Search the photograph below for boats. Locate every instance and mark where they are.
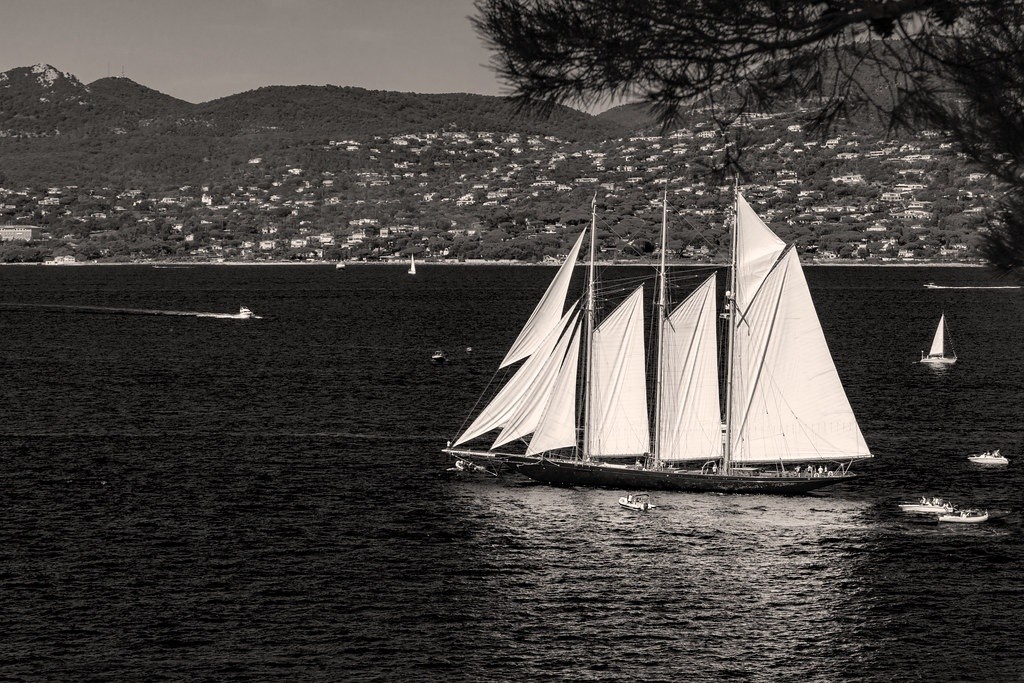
[335,261,347,271]
[238,304,257,319]
[431,350,446,362]
[967,448,1009,466]
[897,504,959,514]
[938,508,990,523]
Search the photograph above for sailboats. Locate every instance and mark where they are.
[407,252,417,275]
[438,171,877,496]
[919,313,958,364]
